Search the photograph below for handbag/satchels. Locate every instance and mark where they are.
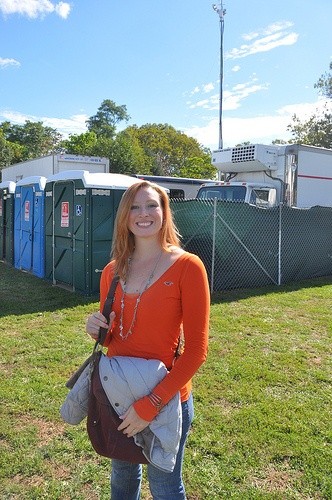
[88,353,147,462]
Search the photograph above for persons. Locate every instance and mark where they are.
[86,181,211,500]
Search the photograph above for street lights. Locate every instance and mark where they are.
[213,0,227,149]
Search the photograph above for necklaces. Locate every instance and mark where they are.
[119,249,163,341]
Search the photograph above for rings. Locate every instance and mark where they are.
[133,429,138,436]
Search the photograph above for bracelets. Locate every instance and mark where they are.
[147,391,165,412]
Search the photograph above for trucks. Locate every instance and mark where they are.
[170,143,332,286]
